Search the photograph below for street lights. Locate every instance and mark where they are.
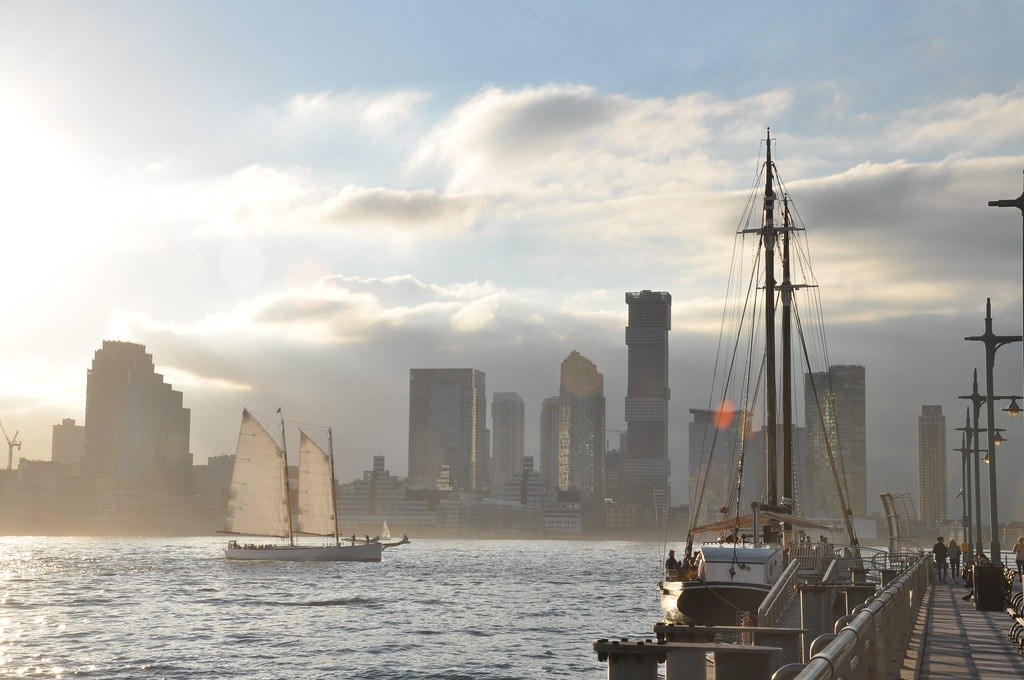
[954,406,1008,557]
[959,367,1024,563]
[951,432,989,555]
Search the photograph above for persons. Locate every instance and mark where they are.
[1012,537,1024,583]
[963,550,995,603]
[665,524,834,586]
[947,538,962,585]
[933,536,947,584]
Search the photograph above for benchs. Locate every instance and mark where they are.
[1006,592,1024,649]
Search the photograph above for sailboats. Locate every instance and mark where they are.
[215,404,414,564]
[648,127,875,627]
[379,519,391,540]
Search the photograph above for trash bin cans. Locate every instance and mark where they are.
[972,565,1004,611]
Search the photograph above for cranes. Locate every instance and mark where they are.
[1,418,24,472]
